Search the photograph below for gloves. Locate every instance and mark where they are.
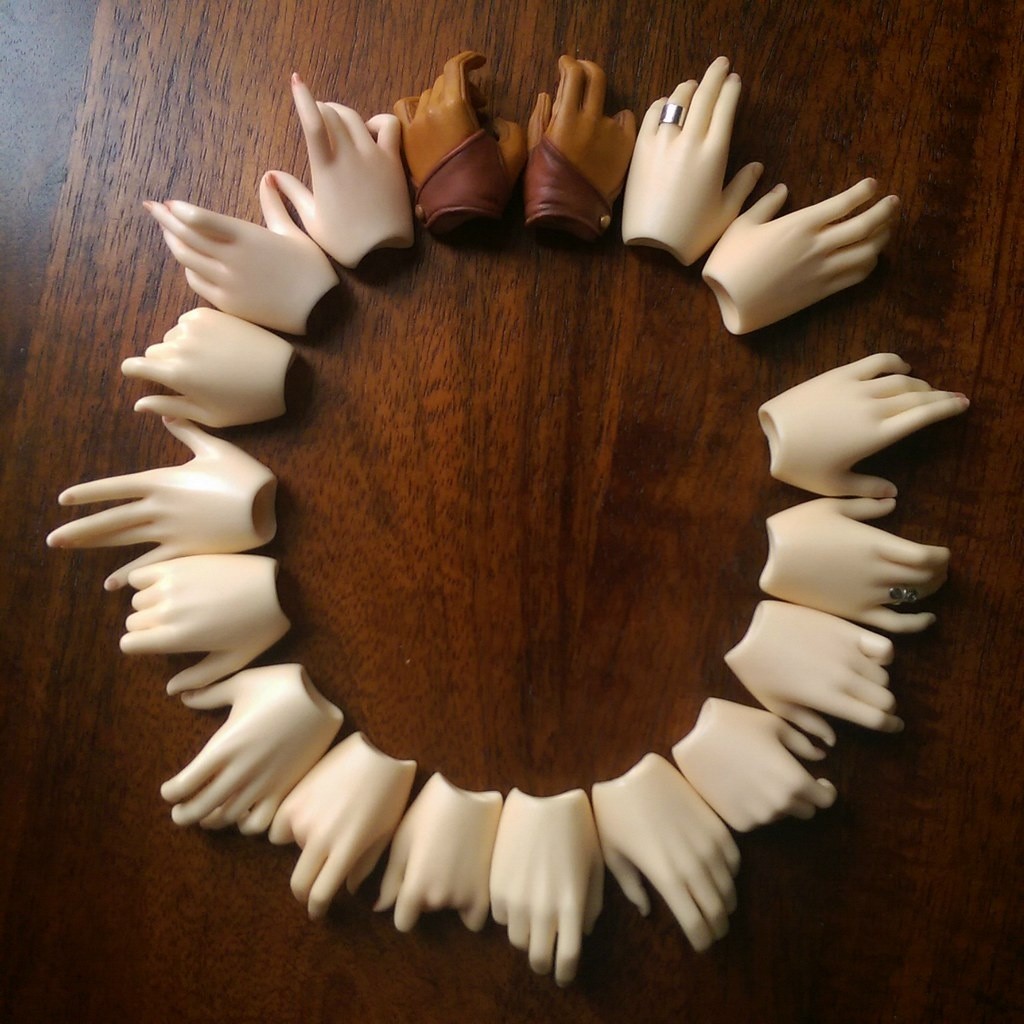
[524,55,636,243]
[393,51,525,245]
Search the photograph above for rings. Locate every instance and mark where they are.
[889,586,920,607]
[657,101,685,128]
